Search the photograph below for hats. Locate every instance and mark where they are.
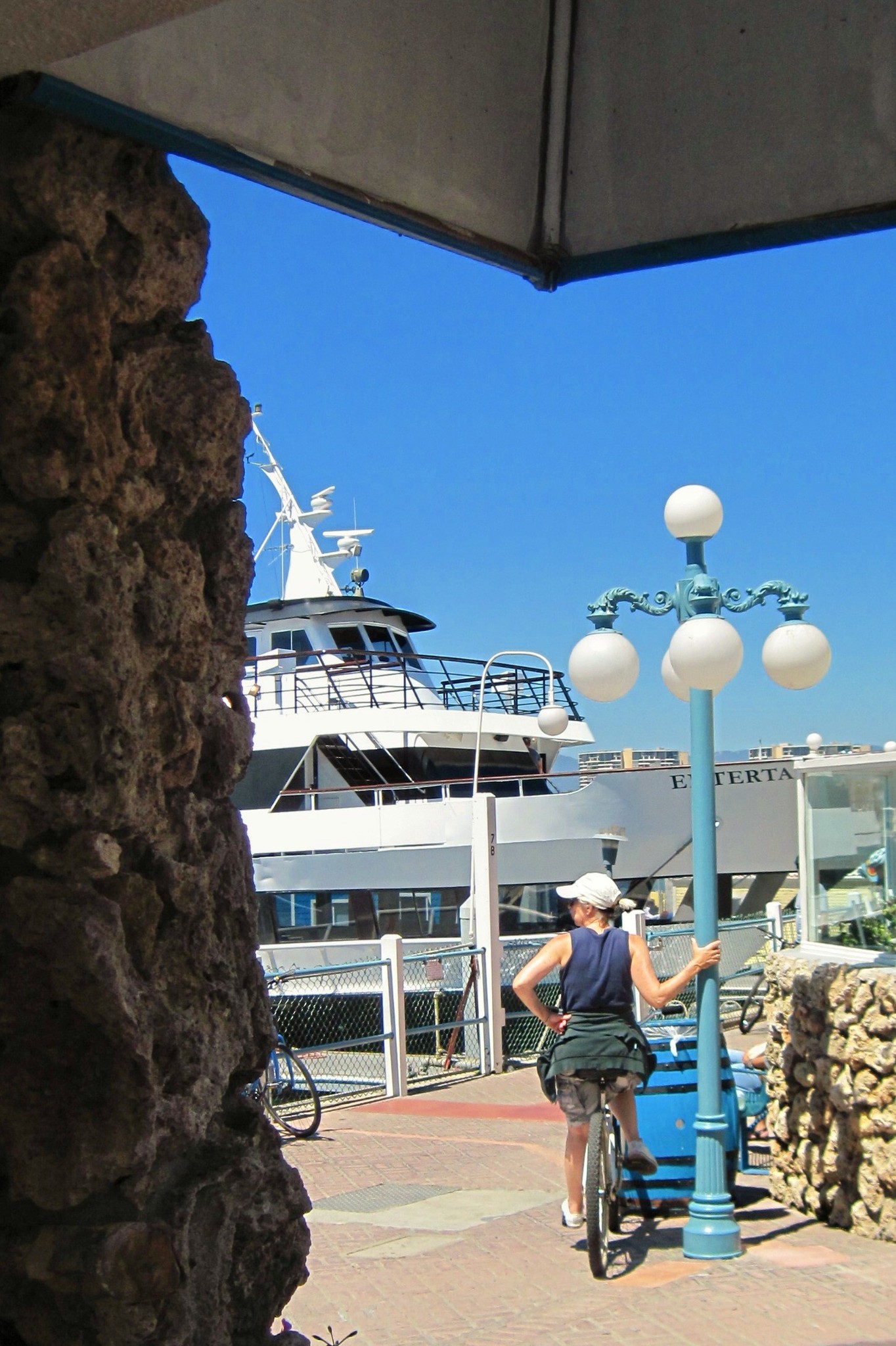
[556,872,622,910]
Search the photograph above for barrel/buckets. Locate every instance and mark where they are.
[617,1018,741,1208]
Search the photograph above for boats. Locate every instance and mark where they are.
[242,400,896,1057]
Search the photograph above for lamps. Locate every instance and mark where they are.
[350,568,370,597]
[495,734,508,742]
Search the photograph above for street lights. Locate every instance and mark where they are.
[565,483,834,1265]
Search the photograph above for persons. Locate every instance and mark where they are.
[643,899,668,920]
[742,1041,769,1140]
[512,872,722,1227]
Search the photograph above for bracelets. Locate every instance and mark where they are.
[748,1059,753,1069]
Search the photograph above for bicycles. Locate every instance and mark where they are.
[739,925,799,1035]
[544,1003,625,1281]
[239,969,322,1140]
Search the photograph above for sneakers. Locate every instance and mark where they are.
[562,1196,584,1227]
[626,1140,658,1175]
